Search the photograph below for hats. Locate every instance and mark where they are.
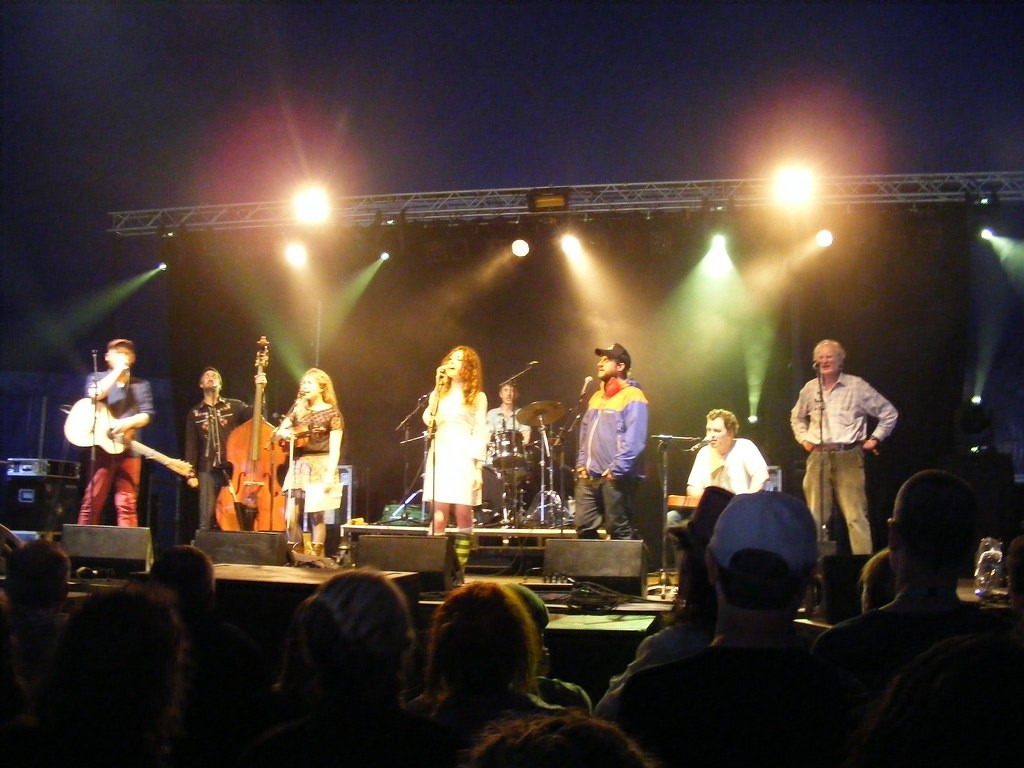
[595,342,631,369]
[710,489,819,571]
[669,484,732,556]
[107,338,134,348]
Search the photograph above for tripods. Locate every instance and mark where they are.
[387,371,575,526]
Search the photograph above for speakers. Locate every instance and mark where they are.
[0,475,77,532]
[60,523,154,576]
[355,534,463,591]
[805,555,878,626]
[543,540,648,598]
[194,528,291,567]
[464,546,544,575]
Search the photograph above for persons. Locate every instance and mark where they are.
[421,345,491,570]
[789,340,900,555]
[572,343,651,541]
[273,366,345,557]
[1,468,1024,768]
[79,338,155,528]
[184,367,269,533]
[667,408,770,568]
[488,379,531,446]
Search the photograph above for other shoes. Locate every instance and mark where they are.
[307,542,323,556]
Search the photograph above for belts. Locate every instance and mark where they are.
[813,443,857,451]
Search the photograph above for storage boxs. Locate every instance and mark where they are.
[7,458,81,479]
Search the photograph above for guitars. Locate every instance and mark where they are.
[63,398,193,477]
[667,495,701,509]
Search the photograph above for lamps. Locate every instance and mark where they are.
[964,194,1001,242]
[157,225,185,272]
[377,224,416,265]
[701,196,734,250]
[510,214,545,258]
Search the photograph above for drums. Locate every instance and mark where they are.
[449,464,506,528]
[486,442,504,475]
[494,429,526,472]
[382,504,431,527]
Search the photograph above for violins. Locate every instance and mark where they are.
[280,423,326,452]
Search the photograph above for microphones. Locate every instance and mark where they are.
[578,375,593,403]
[689,434,714,453]
[813,361,820,370]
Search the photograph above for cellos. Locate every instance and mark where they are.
[214,336,287,532]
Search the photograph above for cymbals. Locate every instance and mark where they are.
[516,401,565,427]
[525,437,562,450]
